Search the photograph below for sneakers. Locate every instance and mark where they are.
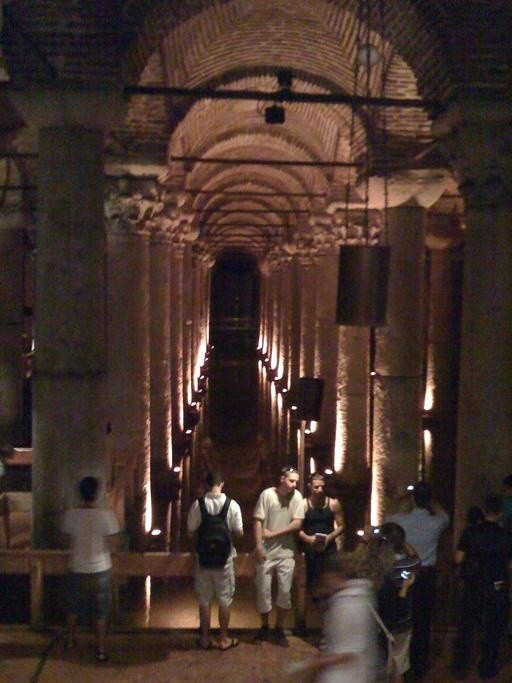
[254,625,288,647]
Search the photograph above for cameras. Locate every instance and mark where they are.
[400,570,409,578]
[372,527,381,534]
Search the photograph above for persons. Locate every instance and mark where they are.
[288,552,374,682]
[383,479,450,672]
[499,473,512,524]
[374,522,423,682]
[297,470,345,647]
[251,464,306,647]
[352,526,413,673]
[56,474,123,664]
[186,468,244,651]
[453,496,511,680]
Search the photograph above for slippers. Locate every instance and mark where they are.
[197,637,238,650]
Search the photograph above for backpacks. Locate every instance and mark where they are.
[196,495,231,568]
